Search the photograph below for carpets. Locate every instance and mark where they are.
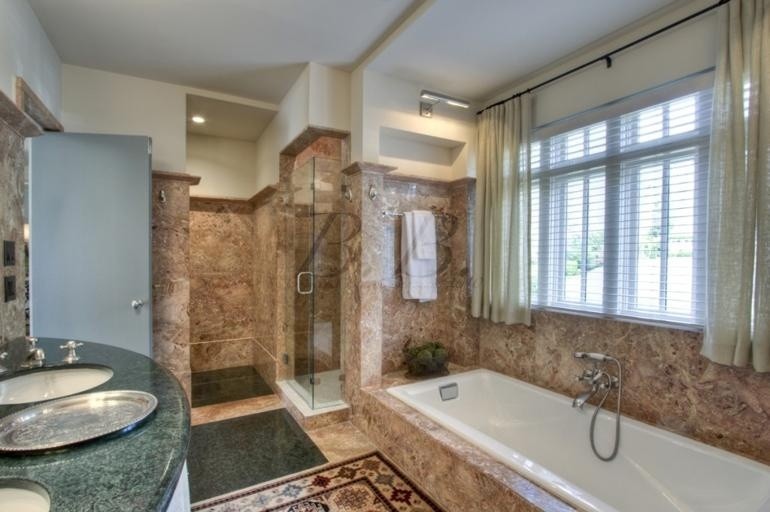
[182,404,451,512]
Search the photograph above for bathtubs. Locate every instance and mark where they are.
[310,319,332,366]
[385,365,770,511]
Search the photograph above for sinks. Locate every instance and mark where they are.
[0,363,115,406]
[0,476,52,512]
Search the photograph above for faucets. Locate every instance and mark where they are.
[21,335,47,368]
[571,381,600,409]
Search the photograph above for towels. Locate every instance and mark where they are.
[399,210,437,302]
